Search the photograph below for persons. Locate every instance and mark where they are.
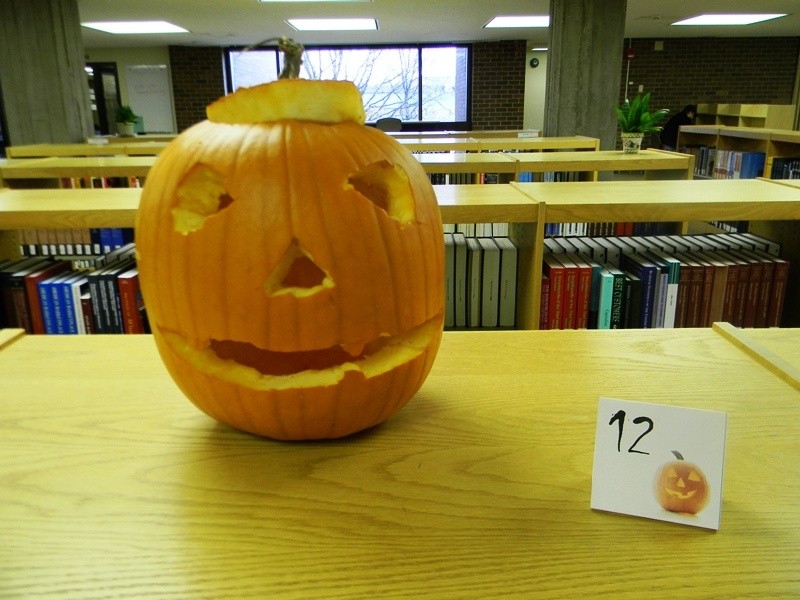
[659,104,697,153]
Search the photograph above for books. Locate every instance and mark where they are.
[0,128,800,336]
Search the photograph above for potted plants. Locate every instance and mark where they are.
[611,90,671,154]
[114,104,141,138]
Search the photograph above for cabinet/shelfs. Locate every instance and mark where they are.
[676,124,800,180]
[381,130,695,237]
[1,325,800,599]
[426,178,800,331]
[0,131,178,264]
[695,102,796,131]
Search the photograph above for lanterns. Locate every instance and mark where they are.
[655,450,709,516]
[132,37,451,442]
